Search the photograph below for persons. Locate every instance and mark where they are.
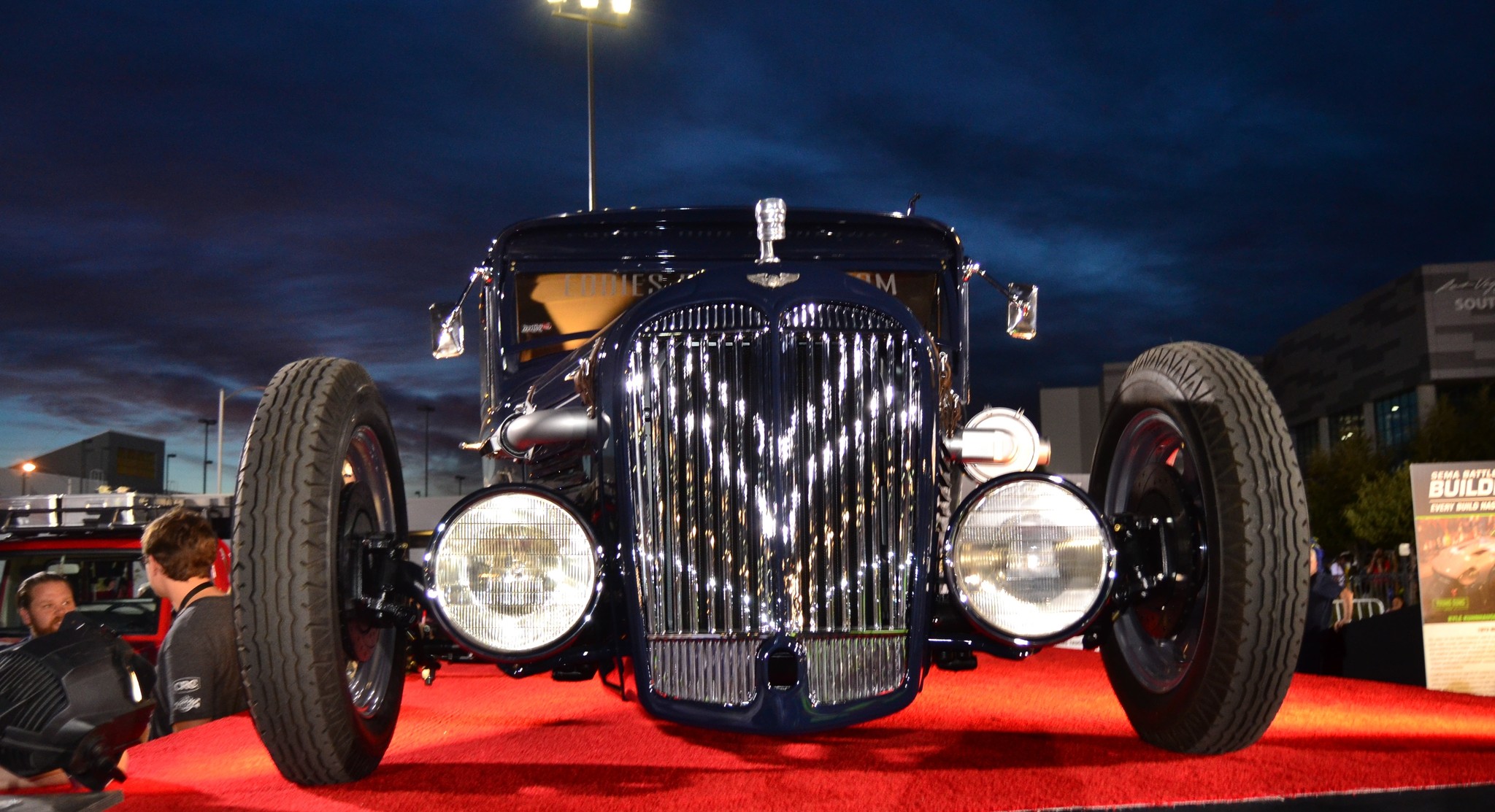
[1302,544,1419,646]
[1,571,77,651]
[141,509,251,742]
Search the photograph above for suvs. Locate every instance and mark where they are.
[0,493,235,679]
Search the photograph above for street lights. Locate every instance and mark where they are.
[165,453,177,492]
[198,417,218,493]
[455,474,466,494]
[21,463,36,496]
[416,404,436,497]
[548,0,632,212]
[79,437,94,494]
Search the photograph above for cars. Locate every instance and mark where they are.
[236,190,1310,788]
